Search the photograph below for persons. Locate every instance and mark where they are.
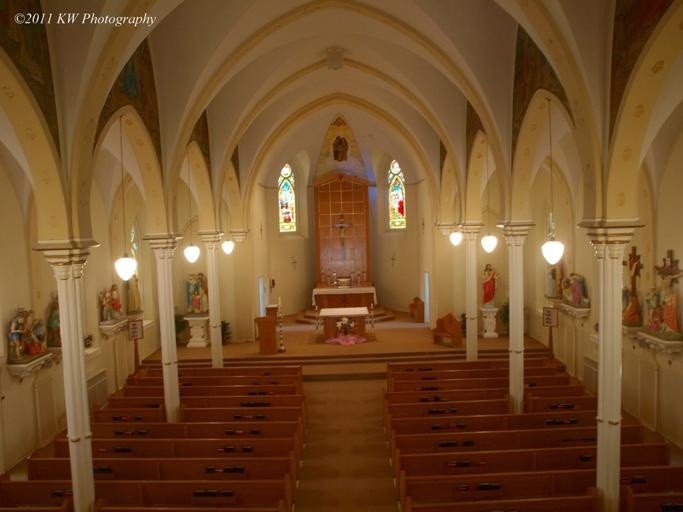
[622,254,682,336]
[481,263,501,308]
[184,272,209,314]
[561,273,586,306]
[6,290,61,363]
[333,225,353,246]
[101,283,124,321]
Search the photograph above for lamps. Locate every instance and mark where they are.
[538,100,564,267]
[183,150,234,266]
[447,142,498,256]
[112,117,137,283]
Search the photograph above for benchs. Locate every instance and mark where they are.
[0,362,310,512]
[381,354,682,511]
